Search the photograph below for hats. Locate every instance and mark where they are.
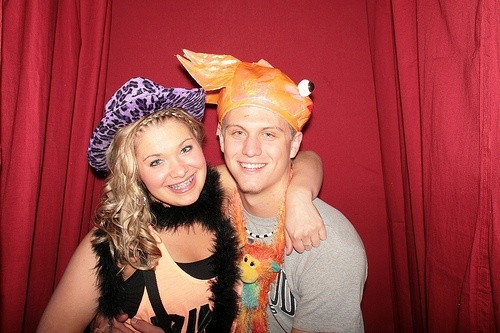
[87,76,207,172]
[176,49,315,132]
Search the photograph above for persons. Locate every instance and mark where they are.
[92,49,368,333]
[37,76,327,333]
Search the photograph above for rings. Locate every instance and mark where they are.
[304,242,312,246]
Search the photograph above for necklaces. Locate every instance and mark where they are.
[244,226,279,239]
